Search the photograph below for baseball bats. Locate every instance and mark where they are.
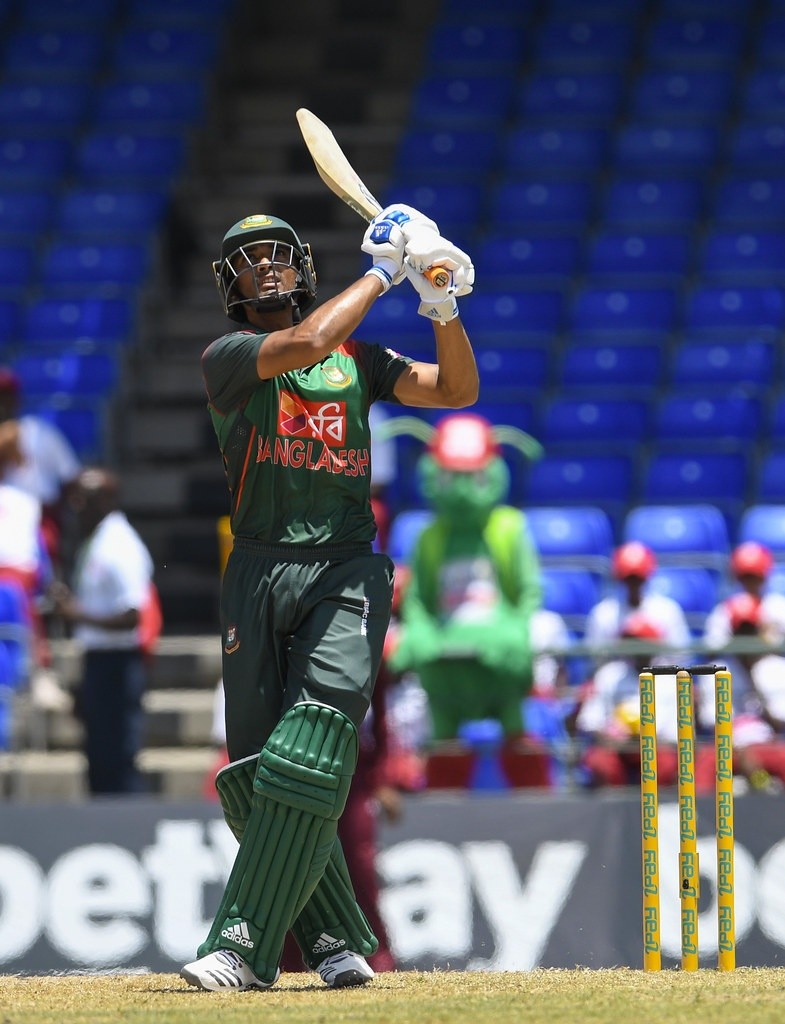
[295,107,452,290]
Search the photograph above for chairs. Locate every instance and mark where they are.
[0,0,784,789]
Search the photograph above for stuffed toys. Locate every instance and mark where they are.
[372,415,543,741]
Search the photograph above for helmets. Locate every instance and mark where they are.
[213,214,317,324]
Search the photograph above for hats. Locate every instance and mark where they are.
[611,540,656,578]
[731,541,774,578]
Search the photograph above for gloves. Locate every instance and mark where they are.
[404,217,475,326]
[360,203,440,297]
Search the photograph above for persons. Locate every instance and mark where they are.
[0,373,164,798]
[176,215,482,993]
[279,537,785,973]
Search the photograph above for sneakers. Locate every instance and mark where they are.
[180,949,282,993]
[316,949,374,986]
